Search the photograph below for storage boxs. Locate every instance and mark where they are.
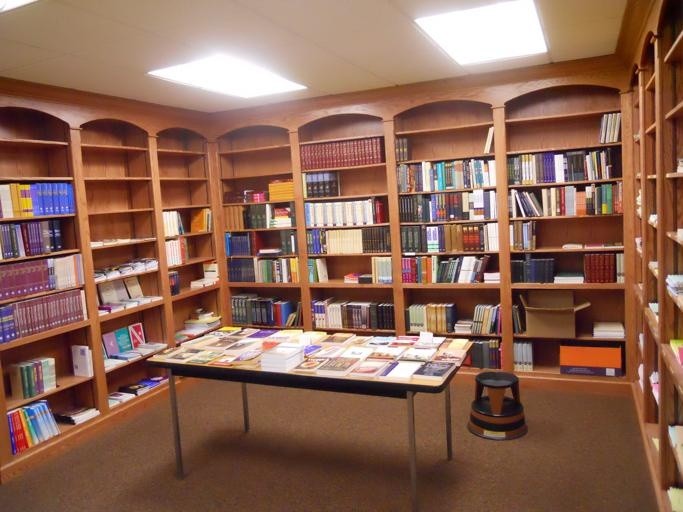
[559,342,622,377]
[519,290,591,339]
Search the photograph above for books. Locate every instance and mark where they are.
[394,127,497,224]
[162,207,220,297]
[506,112,624,282]
[299,137,395,330]
[634,159,683,512]
[592,320,625,338]
[222,179,303,327]
[90,238,169,408]
[400,222,501,336]
[147,308,501,382]
[514,342,533,372]
[0,183,89,344]
[6,345,101,455]
[512,303,523,335]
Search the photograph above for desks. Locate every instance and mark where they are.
[144,327,472,512]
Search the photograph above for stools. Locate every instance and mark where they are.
[467,372,528,441]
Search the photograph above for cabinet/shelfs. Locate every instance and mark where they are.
[210,56,631,397]
[614,0,683,512]
[0,77,227,484]
